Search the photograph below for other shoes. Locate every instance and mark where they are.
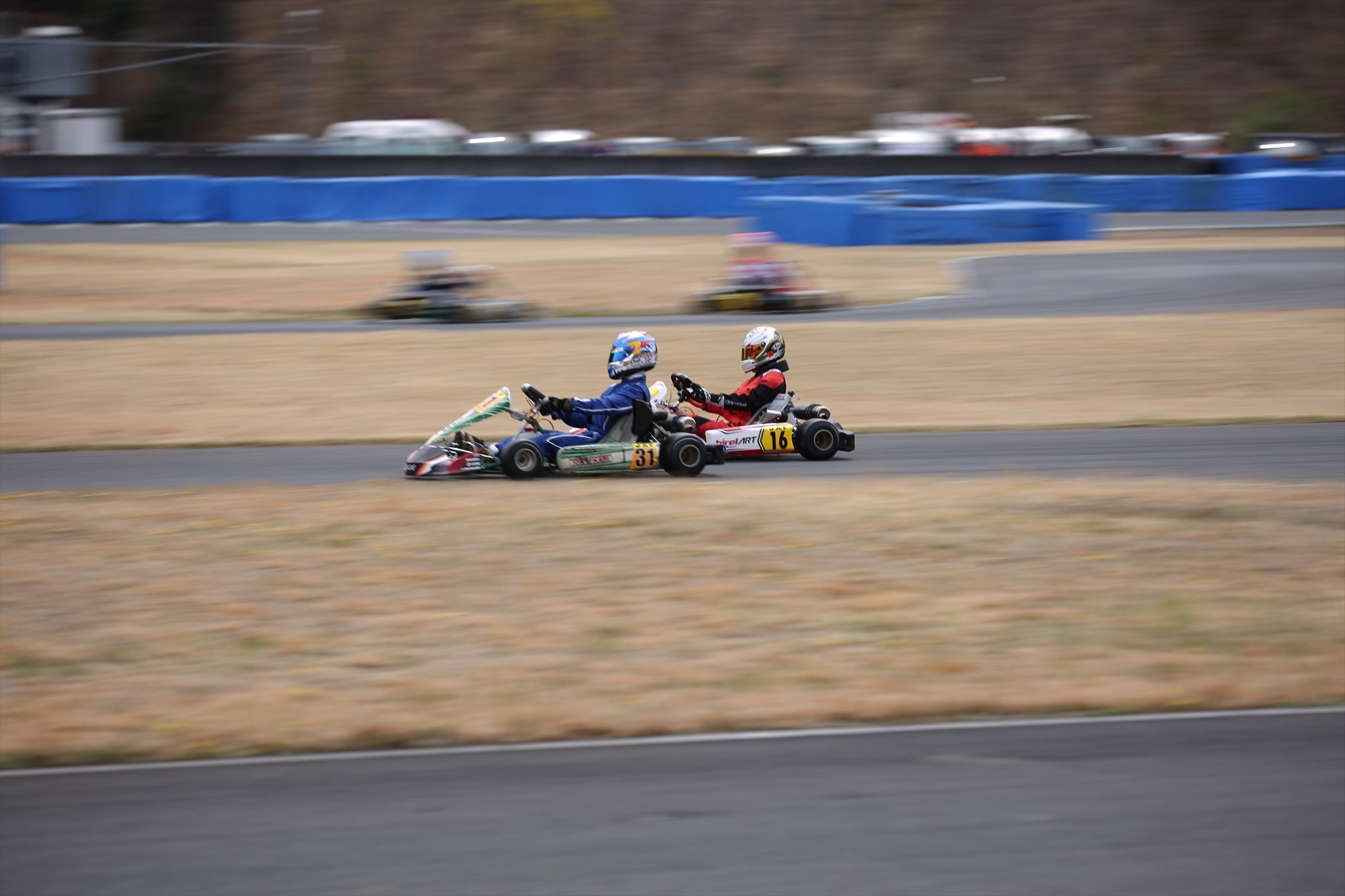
[455,430,468,457]
[465,432,487,455]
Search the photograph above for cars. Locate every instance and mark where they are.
[244,108,1345,160]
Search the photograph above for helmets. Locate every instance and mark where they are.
[740,326,784,373]
[605,330,658,379]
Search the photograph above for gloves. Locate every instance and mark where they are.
[539,396,571,416]
[689,387,711,403]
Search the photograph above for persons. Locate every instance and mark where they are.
[455,330,658,457]
[671,326,789,440]
